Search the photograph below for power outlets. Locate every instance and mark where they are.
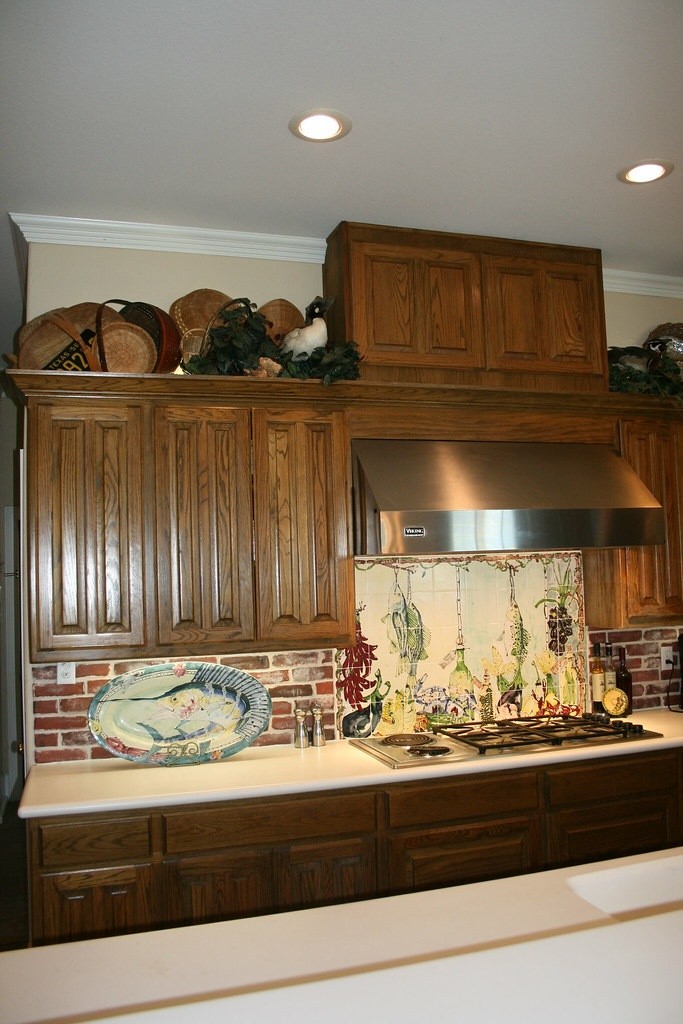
[661,646,673,670]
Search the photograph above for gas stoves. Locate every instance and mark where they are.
[347,712,665,769]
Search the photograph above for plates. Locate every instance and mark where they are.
[91,322,157,373]
[88,661,273,765]
[601,687,629,716]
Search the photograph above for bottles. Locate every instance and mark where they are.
[310,705,326,746]
[590,641,632,714]
[294,708,309,749]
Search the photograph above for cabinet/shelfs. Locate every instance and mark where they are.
[17,223,683,665]
[27,746,683,947]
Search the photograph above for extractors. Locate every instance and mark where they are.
[351,438,666,557]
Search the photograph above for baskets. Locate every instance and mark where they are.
[17,289,304,375]
